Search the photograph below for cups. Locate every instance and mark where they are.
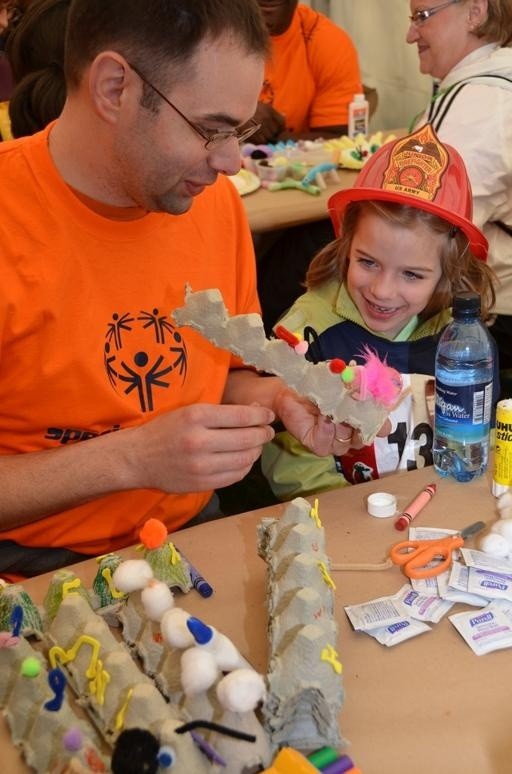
[240,152,316,184]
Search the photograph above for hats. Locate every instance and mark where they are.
[327,124,489,262]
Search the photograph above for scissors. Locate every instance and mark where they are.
[389,521,486,579]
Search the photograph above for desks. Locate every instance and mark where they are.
[221,127,416,239]
[0,460,512,772]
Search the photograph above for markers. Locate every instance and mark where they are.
[395,484,436,531]
[175,547,212,598]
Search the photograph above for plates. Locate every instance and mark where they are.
[227,168,262,197]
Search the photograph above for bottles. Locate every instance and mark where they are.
[432,286,494,481]
[348,91,369,141]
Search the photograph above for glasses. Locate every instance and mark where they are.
[409,0,456,25]
[127,61,262,150]
